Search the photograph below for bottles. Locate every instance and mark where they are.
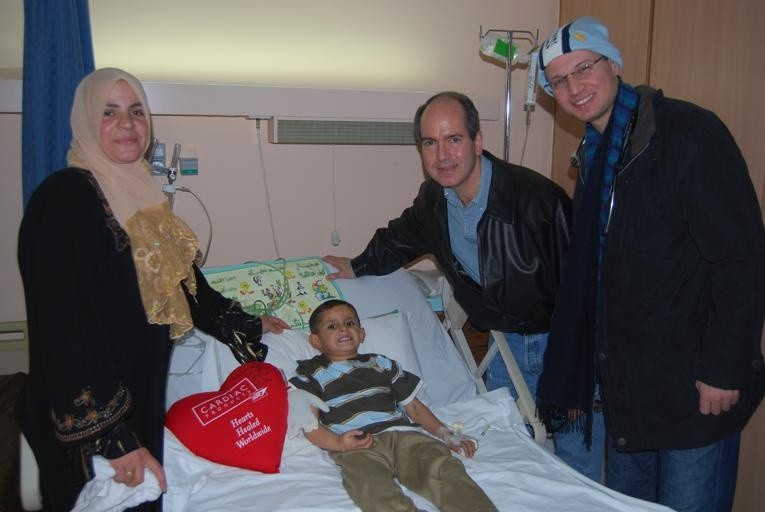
[479,33,530,68]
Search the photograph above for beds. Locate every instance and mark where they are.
[160,260,671,511]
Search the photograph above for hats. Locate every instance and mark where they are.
[537,16,622,96]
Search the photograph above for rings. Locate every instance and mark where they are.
[126,471,133,475]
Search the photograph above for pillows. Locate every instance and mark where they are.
[213,309,430,439]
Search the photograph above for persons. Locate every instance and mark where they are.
[322,93,606,487]
[533,18,764,511]
[14,68,289,511]
[291,300,497,512]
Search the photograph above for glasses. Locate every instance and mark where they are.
[544,57,604,96]
